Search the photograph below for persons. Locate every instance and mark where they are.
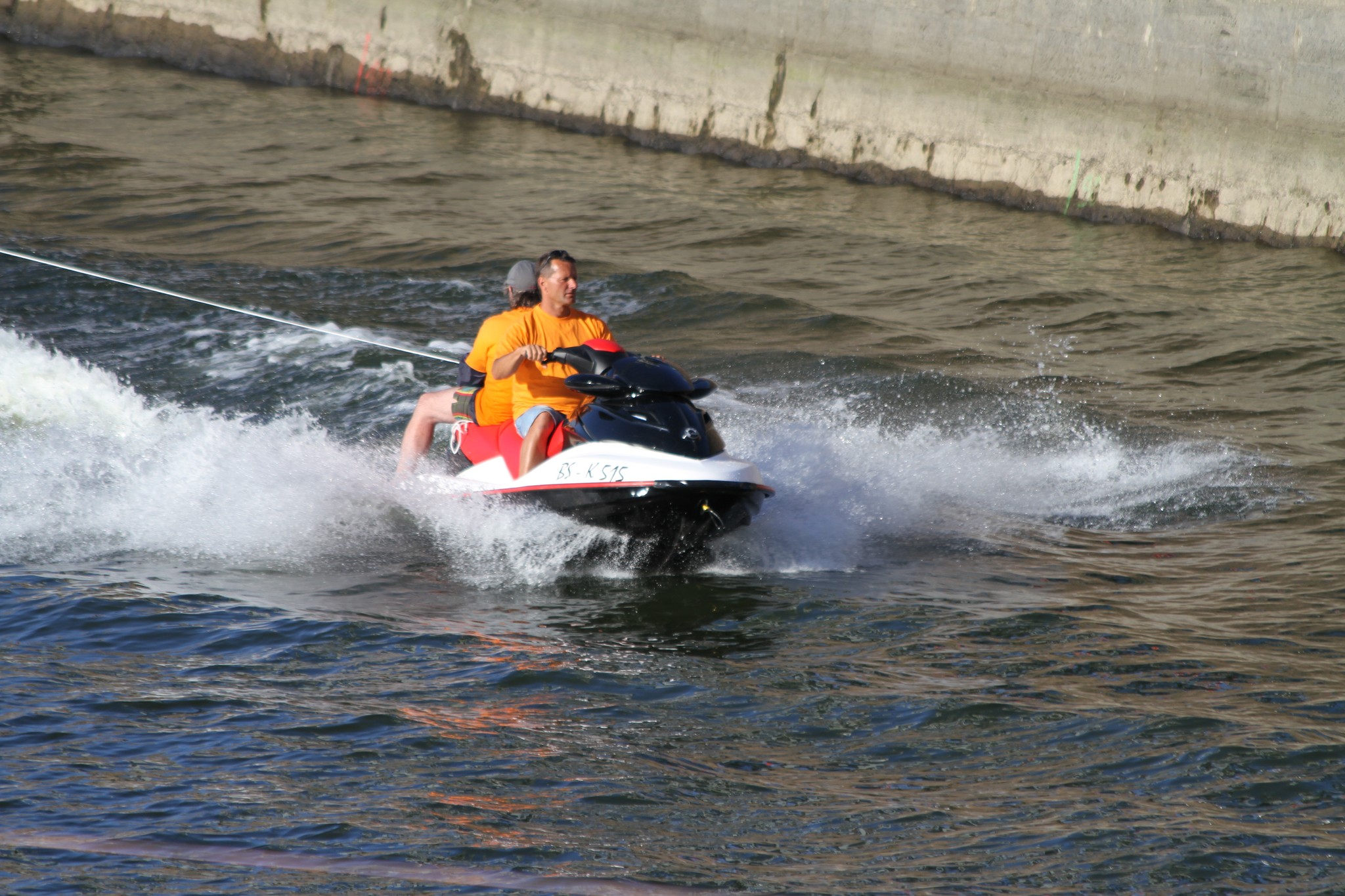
[395,259,543,485]
[487,249,615,481]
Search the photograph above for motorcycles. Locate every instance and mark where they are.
[400,338,780,580]
